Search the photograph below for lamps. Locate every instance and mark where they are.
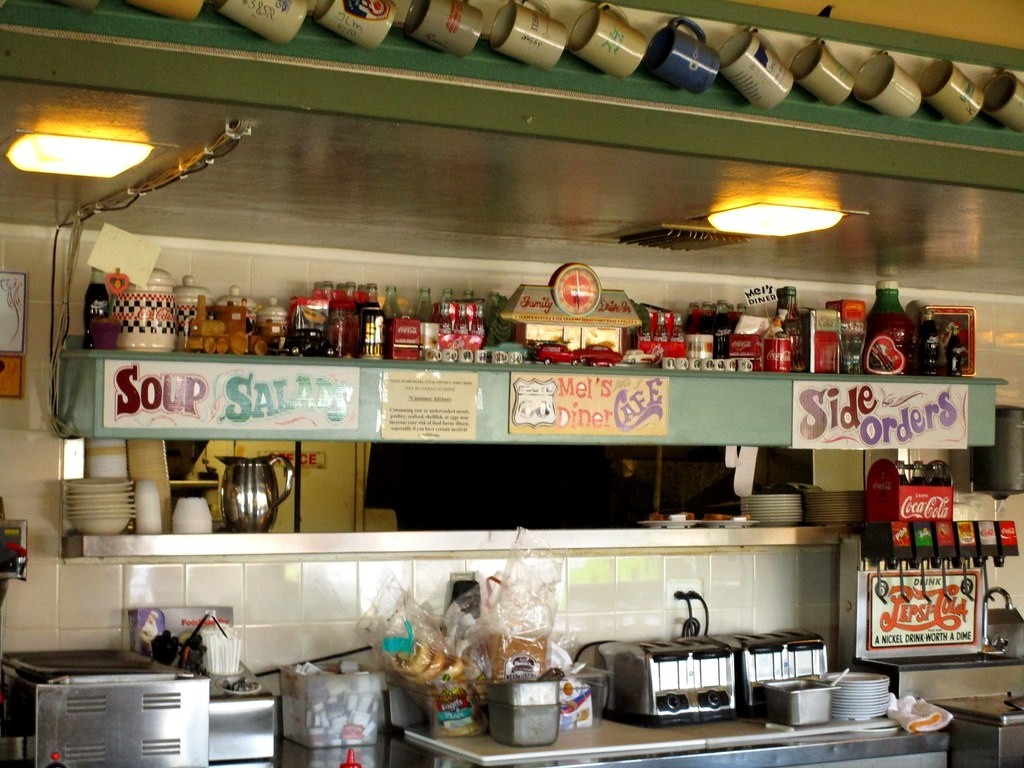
[0,130,178,178]
[685,201,869,238]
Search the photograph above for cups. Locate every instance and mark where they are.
[836,318,867,374]
[662,357,753,372]
[91,320,119,349]
[764,339,792,373]
[426,349,523,364]
[136,481,162,534]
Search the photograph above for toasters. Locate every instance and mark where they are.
[594,628,830,727]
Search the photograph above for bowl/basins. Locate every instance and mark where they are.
[63,438,172,532]
[173,497,213,534]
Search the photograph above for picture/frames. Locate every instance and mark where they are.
[923,306,977,376]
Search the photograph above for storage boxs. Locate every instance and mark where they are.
[127,605,234,658]
[560,680,593,730]
[278,666,384,747]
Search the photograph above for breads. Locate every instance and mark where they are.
[649,512,751,521]
[392,639,486,737]
[478,630,550,683]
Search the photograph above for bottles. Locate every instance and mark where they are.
[437,288,498,333]
[360,283,384,359]
[775,286,806,372]
[863,280,916,376]
[316,281,366,358]
[381,286,402,358]
[113,269,287,353]
[412,288,433,322]
[684,300,748,359]
[946,327,961,377]
[919,311,939,377]
[84,267,110,349]
[896,460,948,486]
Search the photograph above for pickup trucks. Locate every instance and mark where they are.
[623,350,656,365]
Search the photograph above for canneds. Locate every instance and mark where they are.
[358,306,386,360]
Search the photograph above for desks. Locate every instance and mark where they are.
[403,710,901,768]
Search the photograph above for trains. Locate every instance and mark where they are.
[186,295,286,356]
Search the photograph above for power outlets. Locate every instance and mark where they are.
[667,580,704,610]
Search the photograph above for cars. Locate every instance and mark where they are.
[483,343,532,360]
[271,328,336,358]
[532,344,582,366]
[575,344,622,368]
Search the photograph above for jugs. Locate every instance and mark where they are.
[215,455,293,533]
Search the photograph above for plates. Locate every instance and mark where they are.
[822,672,891,722]
[638,490,868,528]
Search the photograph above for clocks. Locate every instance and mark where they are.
[500,262,643,364]
